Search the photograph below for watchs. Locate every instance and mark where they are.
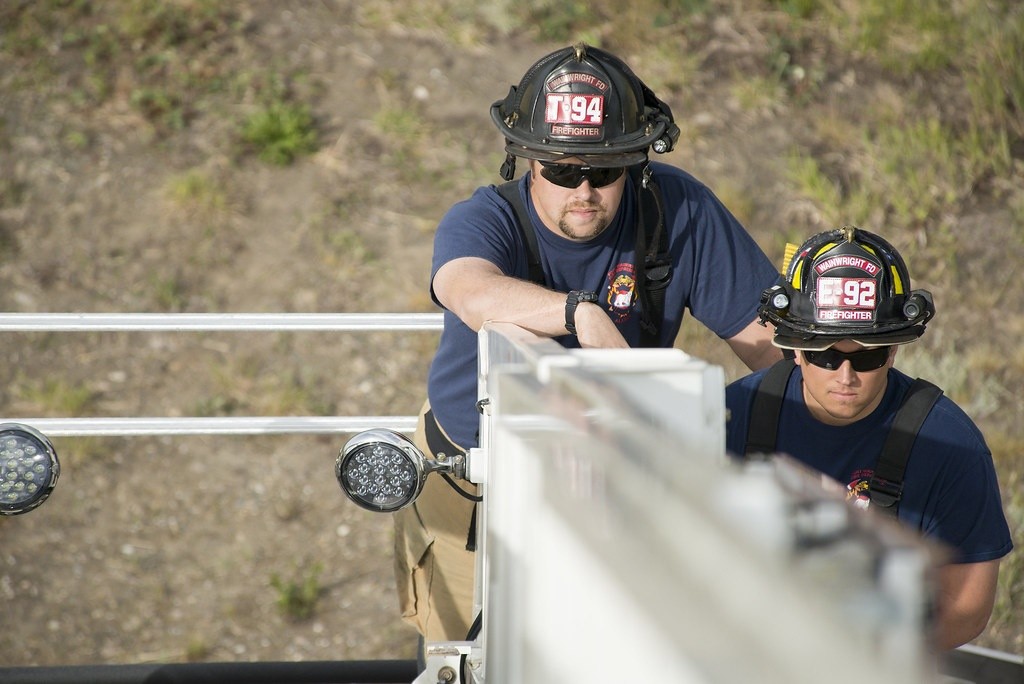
[564,290,598,335]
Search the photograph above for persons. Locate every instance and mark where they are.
[723,226,1014,656]
[396,45,789,684]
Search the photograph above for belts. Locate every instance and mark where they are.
[423,409,467,459]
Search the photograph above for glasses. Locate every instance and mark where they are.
[537,159,625,188]
[803,346,890,373]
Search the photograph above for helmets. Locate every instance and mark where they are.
[757,225,936,352]
[490,42,681,181]
